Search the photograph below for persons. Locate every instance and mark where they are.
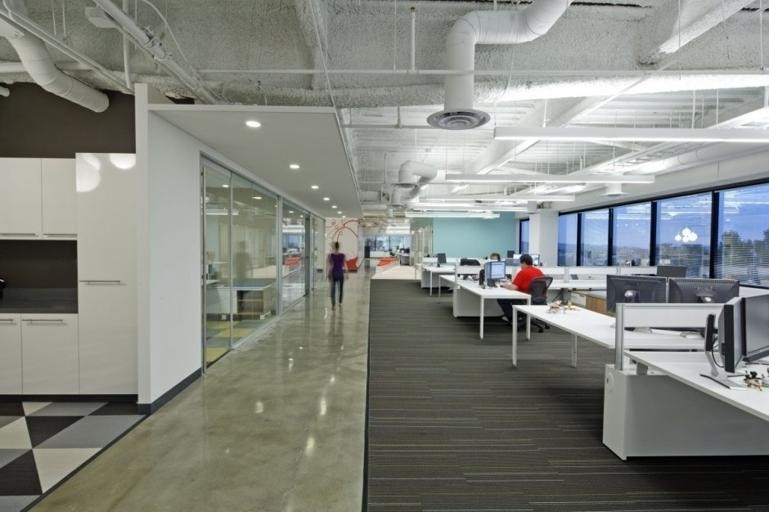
[324,242,349,310]
[233,240,255,311]
[490,253,500,262]
[497,253,545,328]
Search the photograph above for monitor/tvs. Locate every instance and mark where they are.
[607,275,667,335]
[461,250,540,281]
[656,265,687,278]
[437,253,446,267]
[700,297,747,390]
[668,277,740,338]
[742,294,769,357]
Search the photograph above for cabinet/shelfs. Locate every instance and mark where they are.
[0,157,77,241]
[75,152,139,394]
[0,312,79,395]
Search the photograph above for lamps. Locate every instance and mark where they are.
[405,90,768,220]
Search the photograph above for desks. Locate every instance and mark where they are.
[420,255,768,461]
